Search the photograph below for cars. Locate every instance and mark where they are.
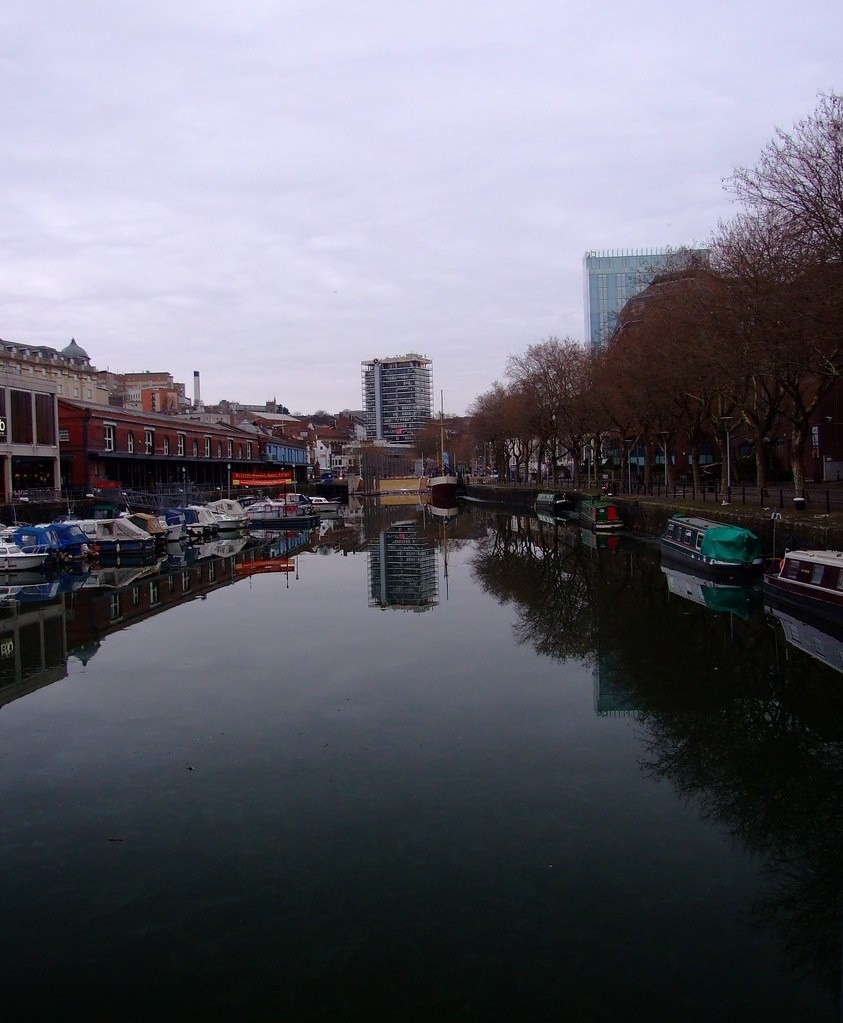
[169,483,197,494]
[321,473,336,484]
[490,470,498,478]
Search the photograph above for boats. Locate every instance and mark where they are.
[532,507,574,525]
[649,550,766,624]
[760,548,843,611]
[0,493,343,571]
[648,511,759,575]
[761,592,843,675]
[579,499,624,532]
[0,521,341,603]
[534,491,574,513]
[577,519,625,552]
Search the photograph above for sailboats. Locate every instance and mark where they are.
[426,408,459,496]
[426,496,461,600]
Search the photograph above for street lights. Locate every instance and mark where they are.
[547,450,552,483]
[586,446,592,490]
[183,467,185,492]
[720,416,737,503]
[228,463,231,498]
[291,462,296,493]
[626,440,635,494]
[657,431,672,497]
